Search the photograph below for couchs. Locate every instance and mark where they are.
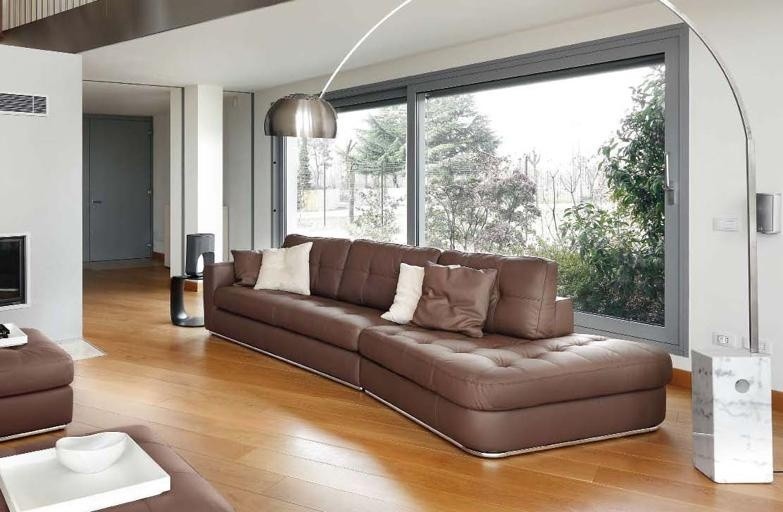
[1,328,75,441]
[2,423,228,512]
[203,228,672,458]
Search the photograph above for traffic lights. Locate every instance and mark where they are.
[230,242,314,295]
[380,258,498,339]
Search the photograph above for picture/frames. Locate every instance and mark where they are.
[691,349,773,486]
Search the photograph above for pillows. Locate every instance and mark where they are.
[230,242,314,295]
[380,258,498,339]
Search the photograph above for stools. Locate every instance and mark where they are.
[171,273,204,327]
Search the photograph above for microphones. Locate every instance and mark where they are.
[171,273,204,327]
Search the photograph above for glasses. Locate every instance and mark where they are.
[263,0,759,354]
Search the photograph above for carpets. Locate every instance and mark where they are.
[84,258,162,272]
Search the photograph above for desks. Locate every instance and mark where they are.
[691,349,773,486]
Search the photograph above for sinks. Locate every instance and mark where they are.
[203,228,672,458]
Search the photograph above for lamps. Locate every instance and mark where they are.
[263,0,759,354]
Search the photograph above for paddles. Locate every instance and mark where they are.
[84,258,162,272]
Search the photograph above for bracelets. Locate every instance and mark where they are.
[55,431,129,474]
